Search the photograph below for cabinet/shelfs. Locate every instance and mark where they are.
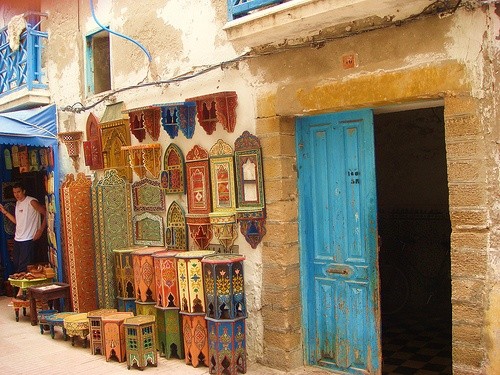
[7,277,51,322]
[28,282,71,334]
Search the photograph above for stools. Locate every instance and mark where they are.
[63,312,90,347]
[37,308,58,339]
[46,311,76,341]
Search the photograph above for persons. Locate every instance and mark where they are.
[0,183,48,307]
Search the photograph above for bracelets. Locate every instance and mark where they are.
[4,211,8,215]
